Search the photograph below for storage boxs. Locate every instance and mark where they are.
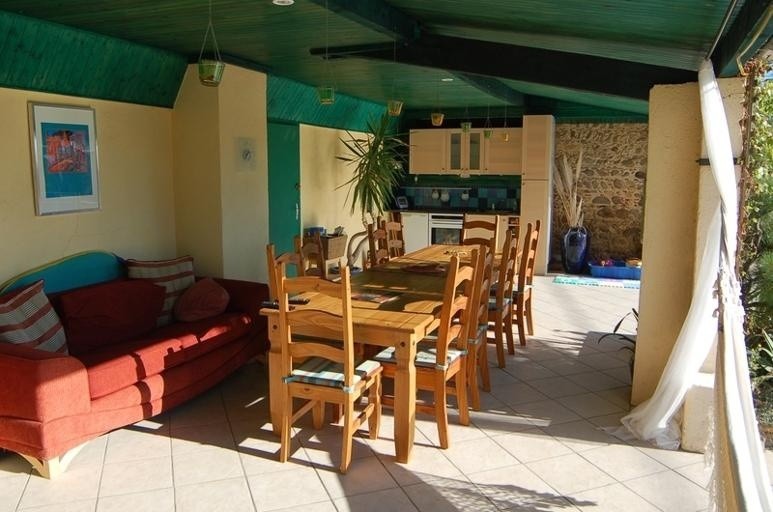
[587,260,634,279]
[302,233,348,260]
[625,262,641,280]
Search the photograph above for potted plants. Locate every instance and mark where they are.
[551,146,591,274]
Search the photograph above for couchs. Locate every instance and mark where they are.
[0,276,271,480]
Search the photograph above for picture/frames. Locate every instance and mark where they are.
[27,100,103,216]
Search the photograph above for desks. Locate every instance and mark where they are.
[258,242,523,464]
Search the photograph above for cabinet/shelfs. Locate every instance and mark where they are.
[309,257,342,282]
[521,115,555,180]
[409,128,523,175]
[384,212,428,255]
[465,214,521,274]
[517,181,553,275]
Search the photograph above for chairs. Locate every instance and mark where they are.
[489,220,541,346]
[370,249,479,449]
[420,238,496,411]
[487,226,522,368]
[460,212,499,250]
[276,261,384,474]
[266,236,307,302]
[377,214,405,264]
[368,220,391,268]
[295,231,326,293]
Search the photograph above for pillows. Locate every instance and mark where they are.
[127,254,196,328]
[47,279,167,353]
[0,278,70,355]
[173,278,230,323]
